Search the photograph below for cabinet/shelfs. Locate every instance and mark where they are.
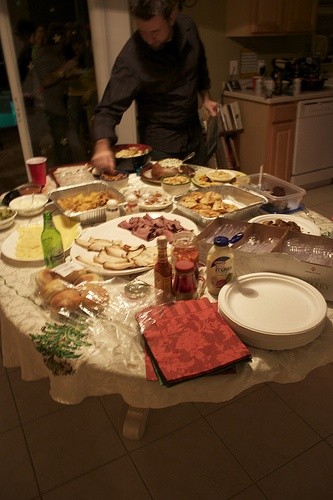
[222,92,333,187]
[225,0,320,37]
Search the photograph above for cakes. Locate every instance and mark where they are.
[151,158,184,178]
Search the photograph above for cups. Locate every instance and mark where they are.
[26,157,49,186]
[253,75,301,96]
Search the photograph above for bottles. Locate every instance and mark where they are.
[40,212,65,269]
[153,230,243,303]
[126,195,139,217]
[106,200,120,222]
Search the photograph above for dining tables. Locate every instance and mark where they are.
[0,158,333,438]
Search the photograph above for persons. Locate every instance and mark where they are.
[24,34,85,163]
[92,0,221,175]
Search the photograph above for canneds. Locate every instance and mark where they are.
[172,232,201,284]
[292,78,301,96]
[252,75,264,95]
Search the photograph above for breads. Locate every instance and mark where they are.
[178,192,240,221]
[34,270,111,315]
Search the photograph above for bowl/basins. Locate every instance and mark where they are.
[170,184,269,228]
[112,143,153,171]
[150,158,183,178]
[46,180,131,226]
[236,172,306,212]
[101,169,129,188]
[0,212,18,231]
[9,194,50,217]
[207,171,235,183]
[161,174,191,196]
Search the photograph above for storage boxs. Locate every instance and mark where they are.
[191,214,333,301]
[240,171,305,210]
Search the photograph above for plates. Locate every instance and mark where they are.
[69,211,196,276]
[142,165,197,183]
[1,220,81,262]
[247,214,321,239]
[192,169,250,188]
[120,184,173,209]
[217,272,327,351]
